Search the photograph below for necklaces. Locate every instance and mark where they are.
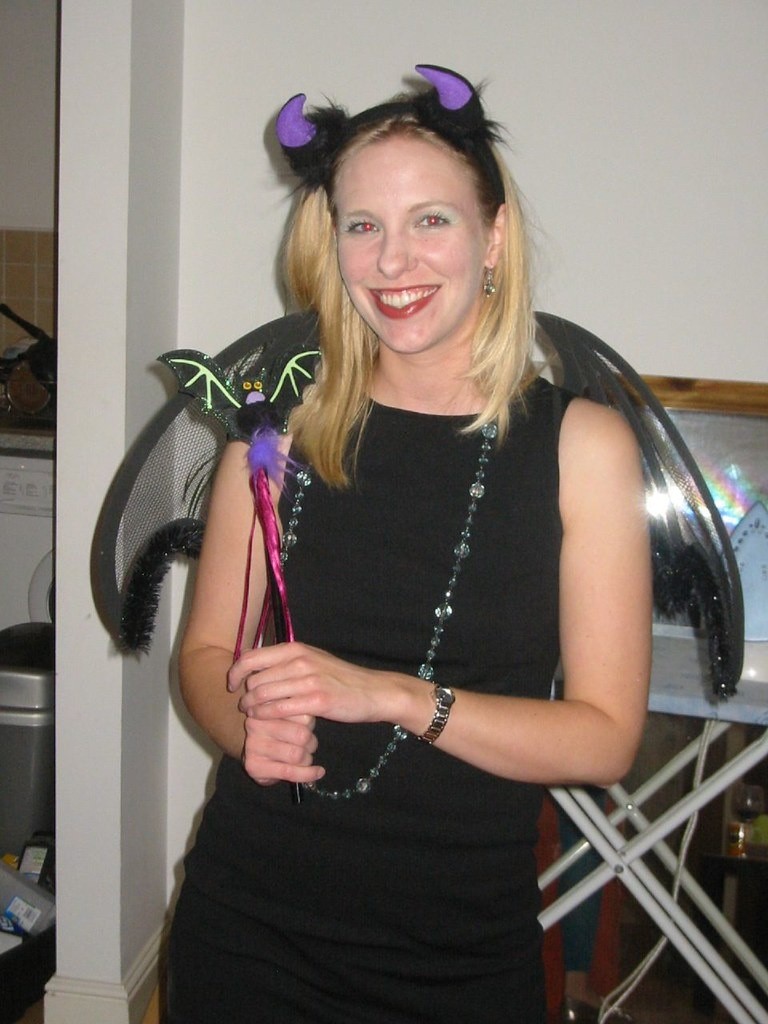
[277,420,499,800]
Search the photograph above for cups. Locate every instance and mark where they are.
[727,822,748,855]
[733,784,765,822]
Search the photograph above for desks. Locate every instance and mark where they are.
[538,635,768,1024]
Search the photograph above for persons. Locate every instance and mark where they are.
[158,64,654,1024]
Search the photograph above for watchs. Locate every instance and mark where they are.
[412,681,456,745]
